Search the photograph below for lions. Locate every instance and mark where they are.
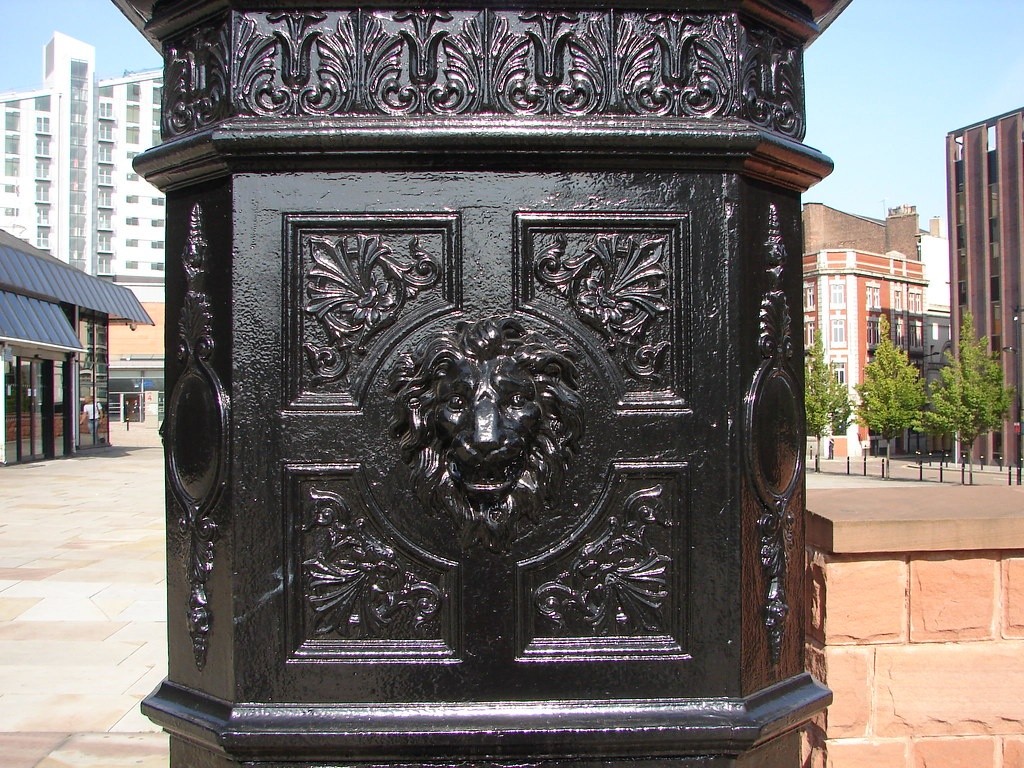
[380,314,587,557]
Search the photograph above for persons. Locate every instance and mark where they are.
[828,438,834,459]
[79,394,103,443]
